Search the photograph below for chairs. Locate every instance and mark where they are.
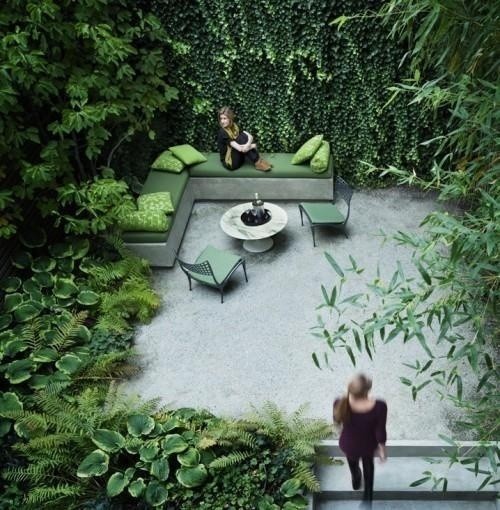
[298,176,355,249]
[171,243,248,303]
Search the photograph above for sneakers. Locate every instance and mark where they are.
[255,159,272,171]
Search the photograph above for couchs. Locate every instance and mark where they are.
[117,151,334,269]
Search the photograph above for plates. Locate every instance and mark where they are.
[240,207,273,227]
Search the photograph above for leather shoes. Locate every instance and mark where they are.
[361,498,372,509]
[352,468,362,490]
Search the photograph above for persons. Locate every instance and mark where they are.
[217,106,272,171]
[332,372,387,510]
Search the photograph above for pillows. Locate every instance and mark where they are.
[117,209,170,233]
[150,149,186,174]
[169,143,208,167]
[135,192,174,214]
[309,139,330,173]
[291,134,324,164]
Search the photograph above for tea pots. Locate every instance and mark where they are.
[251,192,267,220]
